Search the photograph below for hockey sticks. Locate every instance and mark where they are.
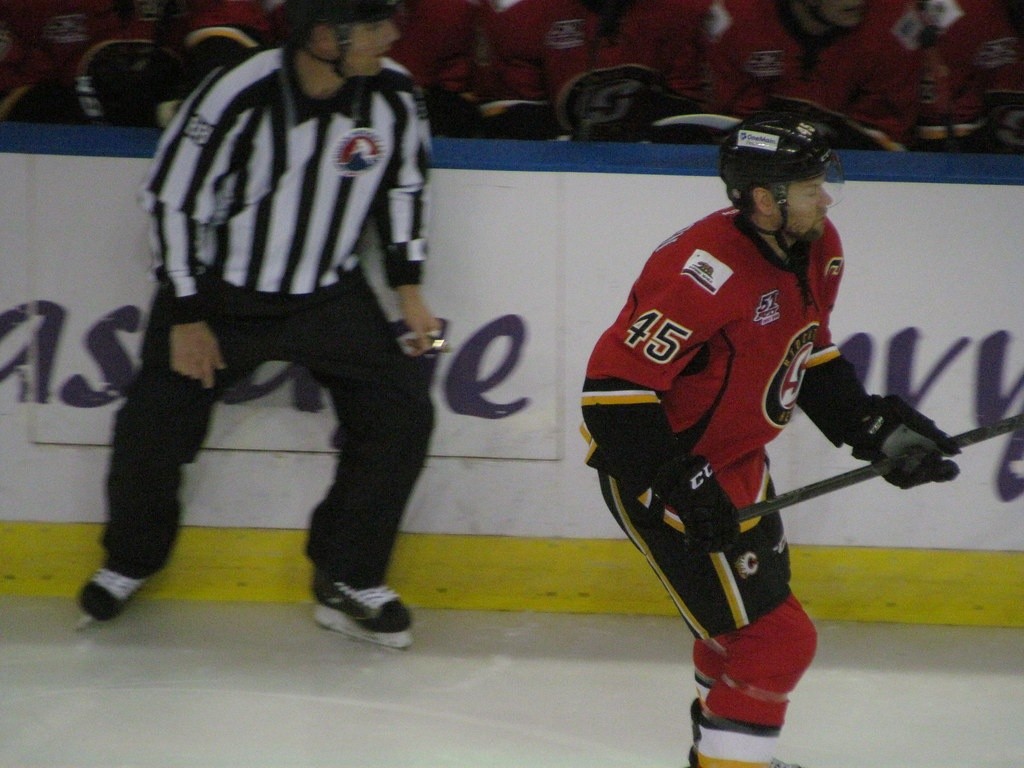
[735,408,1022,523]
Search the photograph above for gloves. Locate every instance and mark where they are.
[654,455,739,555]
[844,394,959,490]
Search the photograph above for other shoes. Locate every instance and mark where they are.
[770,757,802,768]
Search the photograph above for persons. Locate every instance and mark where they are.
[75,1,449,645]
[581,101,962,768]
[0,0,1024,161]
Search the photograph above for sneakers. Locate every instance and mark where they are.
[313,568,412,647]
[77,558,149,628]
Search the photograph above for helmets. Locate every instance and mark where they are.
[262,0,396,49]
[720,113,832,210]
